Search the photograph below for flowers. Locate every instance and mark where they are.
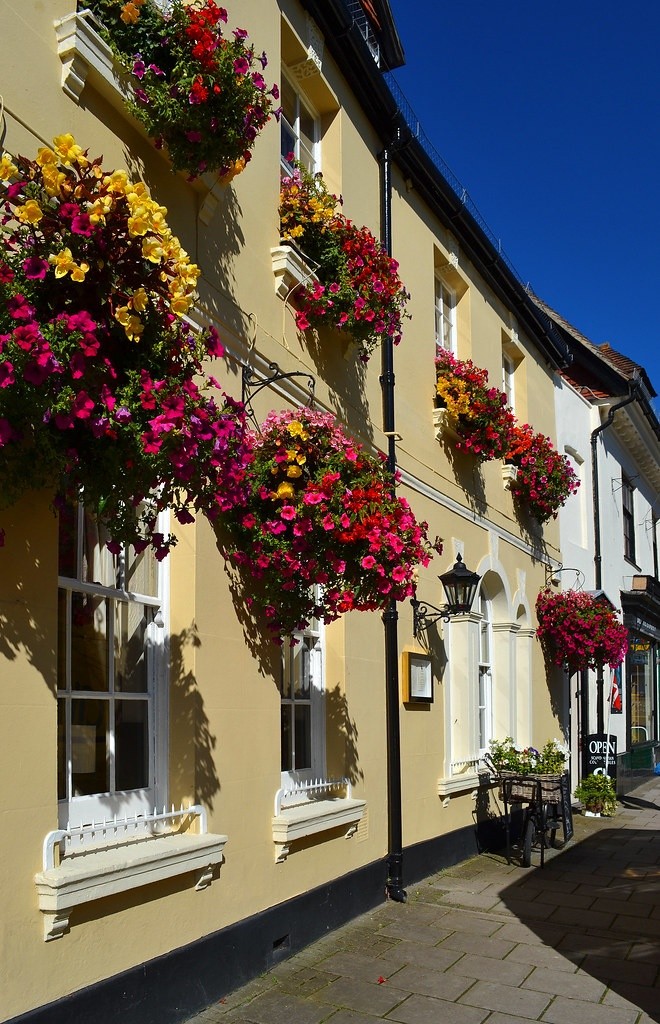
[506,423,582,525]
[433,345,518,460]
[208,405,445,645]
[0,132,261,552]
[75,0,286,188]
[277,152,413,361]
[537,584,631,677]
[487,734,573,778]
[573,773,620,817]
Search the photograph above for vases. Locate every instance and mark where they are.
[586,801,606,813]
[497,768,564,804]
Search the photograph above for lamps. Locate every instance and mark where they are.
[412,551,481,638]
[544,562,617,612]
